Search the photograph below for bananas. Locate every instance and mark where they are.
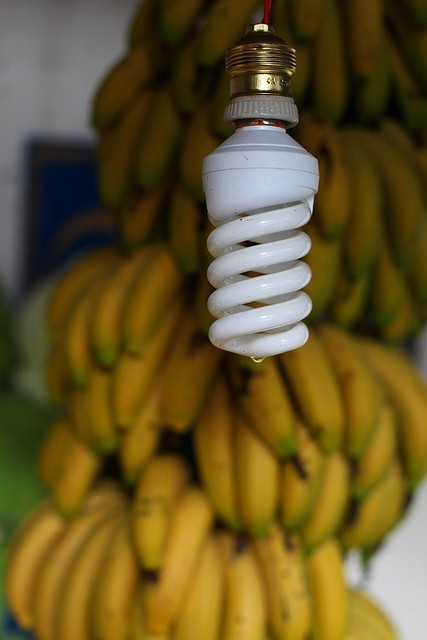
[5,0,427,638]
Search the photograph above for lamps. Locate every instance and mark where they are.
[201,0,321,362]
